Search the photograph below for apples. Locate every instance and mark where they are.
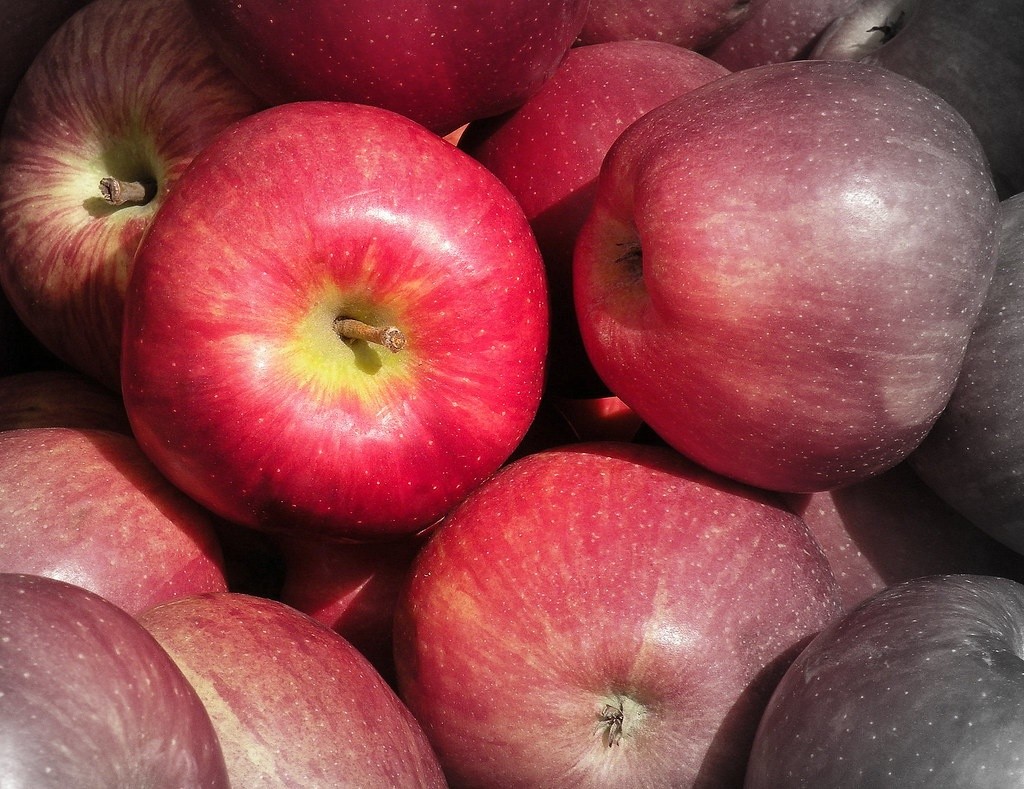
[0,0,1024,789]
